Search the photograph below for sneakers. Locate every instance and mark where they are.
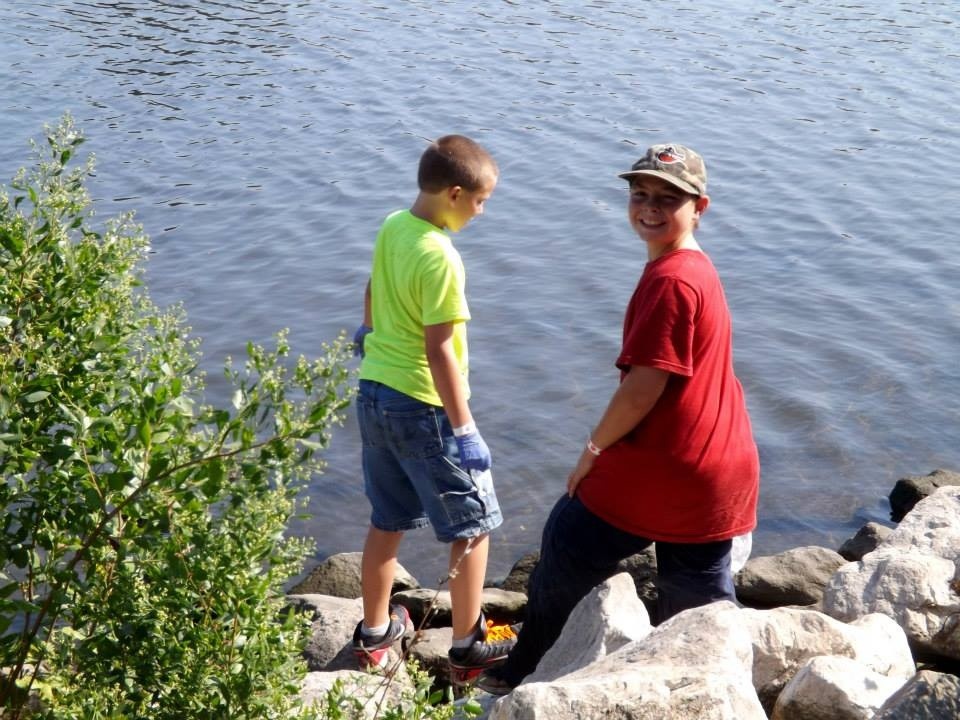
[448,610,522,687]
[353,603,409,673]
[478,669,514,696]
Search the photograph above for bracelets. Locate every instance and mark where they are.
[586,439,602,455]
[452,421,478,436]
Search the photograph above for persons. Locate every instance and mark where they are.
[481,142,760,693]
[351,133,524,680]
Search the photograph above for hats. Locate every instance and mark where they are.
[617,143,707,198]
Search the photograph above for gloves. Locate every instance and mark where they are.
[354,324,374,360]
[455,428,492,473]
